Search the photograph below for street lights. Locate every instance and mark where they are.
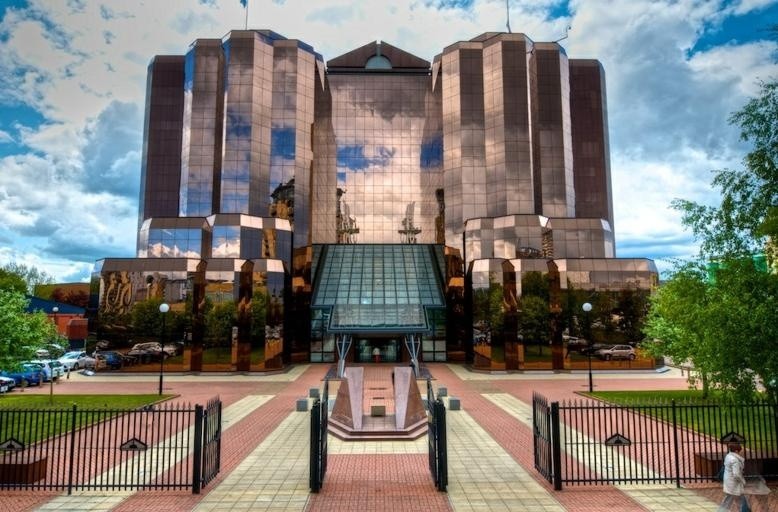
[52,306,59,323]
[159,303,169,395]
[582,302,593,392]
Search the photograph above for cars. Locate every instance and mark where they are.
[98,341,184,368]
[0,342,85,393]
[566,336,636,361]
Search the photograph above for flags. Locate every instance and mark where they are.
[240,0,248,8]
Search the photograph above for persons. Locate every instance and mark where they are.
[719,442,751,512]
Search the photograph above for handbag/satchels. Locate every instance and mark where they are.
[717,466,725,482]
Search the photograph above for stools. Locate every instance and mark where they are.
[437,384,448,396]
[310,388,318,398]
[296,398,308,411]
[449,398,461,410]
[370,402,386,417]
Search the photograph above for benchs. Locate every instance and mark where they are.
[1,454,46,484]
[694,448,778,482]
